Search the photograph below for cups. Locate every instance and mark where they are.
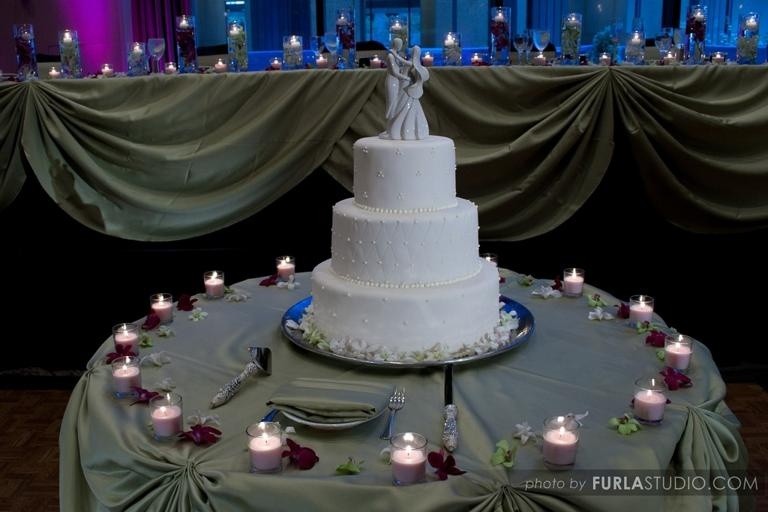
[110,356,143,400]
[10,2,760,82]
[149,392,184,440]
[203,269,226,300]
[275,255,297,284]
[633,376,669,427]
[664,332,694,375]
[542,414,582,472]
[389,432,430,486]
[246,422,285,474]
[480,252,502,275]
[149,292,174,324]
[563,267,587,299]
[629,293,655,330]
[111,323,140,359]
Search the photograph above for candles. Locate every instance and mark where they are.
[106,253,691,482]
[12,7,760,77]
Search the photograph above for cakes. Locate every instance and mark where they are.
[299,38,512,361]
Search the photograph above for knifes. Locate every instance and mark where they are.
[441,362,461,454]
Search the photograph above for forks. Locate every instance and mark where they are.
[383,383,407,441]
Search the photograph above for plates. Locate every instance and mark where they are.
[276,294,537,374]
[279,403,390,434]
